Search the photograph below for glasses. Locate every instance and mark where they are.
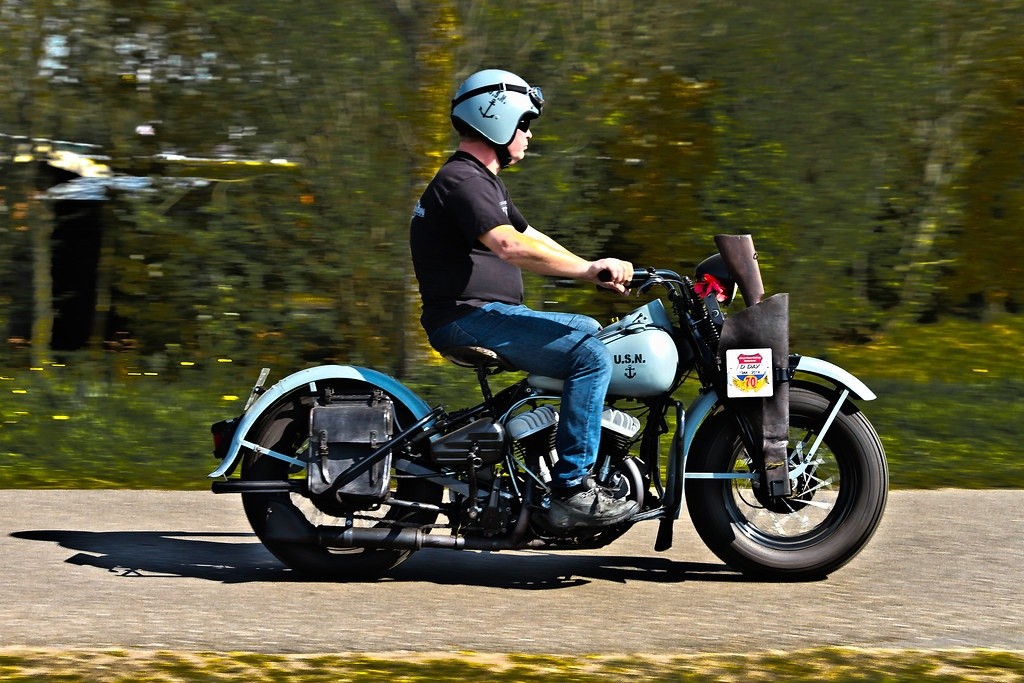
[517,115,530,132]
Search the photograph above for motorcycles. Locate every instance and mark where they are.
[206,233,891,584]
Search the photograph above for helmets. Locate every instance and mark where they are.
[450,69,539,147]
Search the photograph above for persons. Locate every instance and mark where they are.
[410,69,641,527]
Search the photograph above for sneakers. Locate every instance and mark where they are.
[549,474,641,528]
